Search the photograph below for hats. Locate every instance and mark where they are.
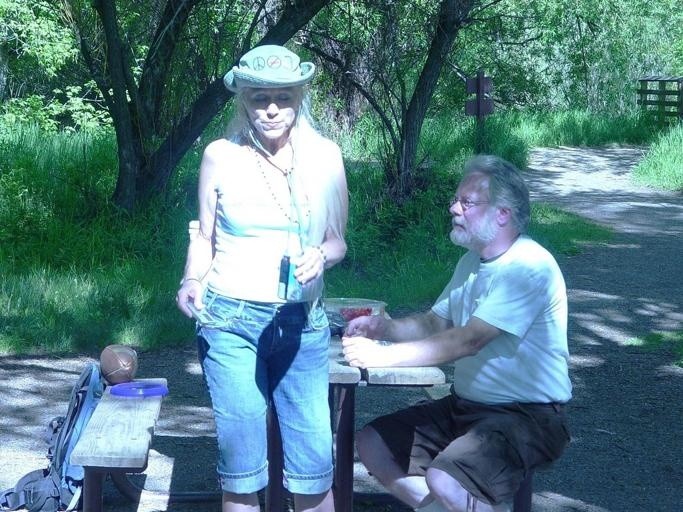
[224,44,316,94]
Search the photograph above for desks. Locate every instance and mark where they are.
[261,299,445,512]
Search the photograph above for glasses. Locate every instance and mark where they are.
[449,194,490,209]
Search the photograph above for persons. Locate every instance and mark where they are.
[339,152,571,512]
[173,43,350,511]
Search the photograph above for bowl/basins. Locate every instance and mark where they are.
[321,298,385,328]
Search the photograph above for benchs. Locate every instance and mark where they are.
[420,382,563,512]
[69,378,167,512]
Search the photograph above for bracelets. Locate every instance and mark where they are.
[311,244,327,265]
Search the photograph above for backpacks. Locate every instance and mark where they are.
[46,359,104,497]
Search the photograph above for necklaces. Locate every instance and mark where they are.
[248,128,311,224]
